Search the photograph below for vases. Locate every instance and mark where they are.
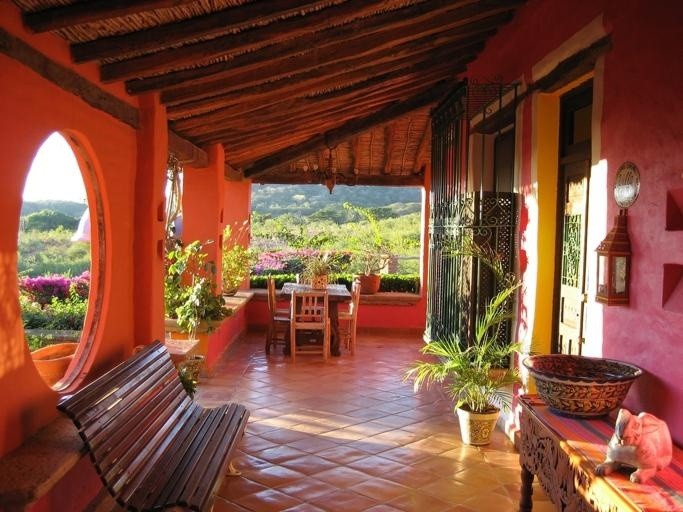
[31,341,79,388]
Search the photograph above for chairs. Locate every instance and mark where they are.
[265,273,362,364]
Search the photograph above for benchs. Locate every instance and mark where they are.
[514,393,683,512]
[55,339,251,512]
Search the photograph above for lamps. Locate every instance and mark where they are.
[323,148,337,195]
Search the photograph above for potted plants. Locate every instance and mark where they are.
[222,216,267,297]
[301,247,353,290]
[174,283,208,384]
[398,279,544,445]
